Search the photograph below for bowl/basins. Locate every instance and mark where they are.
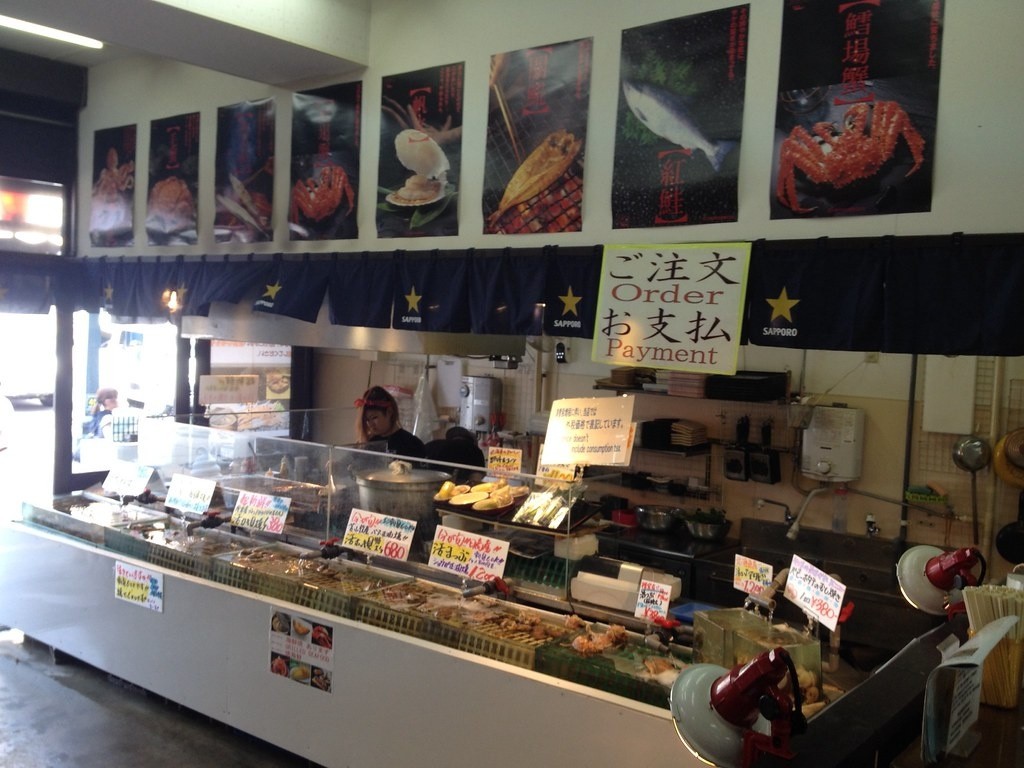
[684,516,731,540]
[633,504,685,532]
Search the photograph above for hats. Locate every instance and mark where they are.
[446,427,478,447]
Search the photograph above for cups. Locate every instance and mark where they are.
[294,456,308,482]
[968,630,1023,706]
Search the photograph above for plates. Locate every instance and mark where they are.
[385,189,446,206]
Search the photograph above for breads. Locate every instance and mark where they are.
[437,477,529,509]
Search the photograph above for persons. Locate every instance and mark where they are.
[91,387,120,442]
[323,385,430,477]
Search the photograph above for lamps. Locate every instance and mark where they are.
[896,544,987,625]
[667,646,808,768]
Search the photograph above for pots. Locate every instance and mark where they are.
[356,469,452,540]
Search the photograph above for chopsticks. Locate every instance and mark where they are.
[962,585,1024,707]
